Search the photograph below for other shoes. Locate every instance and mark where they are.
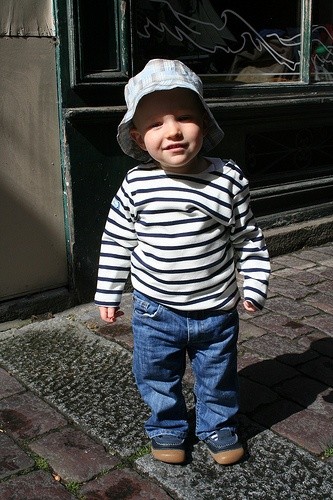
[150,435,185,463]
[205,428,245,465]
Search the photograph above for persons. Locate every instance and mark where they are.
[93,57,272,466]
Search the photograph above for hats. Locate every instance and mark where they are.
[115,58,225,162]
